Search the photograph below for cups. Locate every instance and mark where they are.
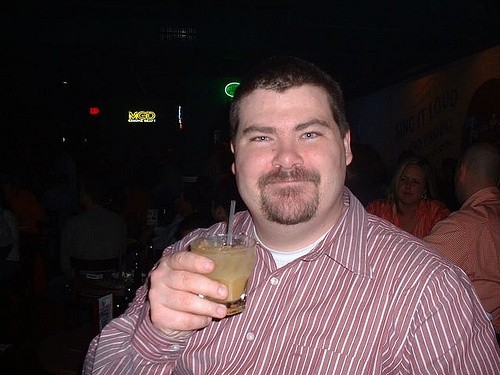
[189,234,257,316]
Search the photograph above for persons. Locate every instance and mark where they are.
[362,157,454,241]
[421,143,500,339]
[81,56,500,375]
[0,144,247,305]
[343,159,372,208]
